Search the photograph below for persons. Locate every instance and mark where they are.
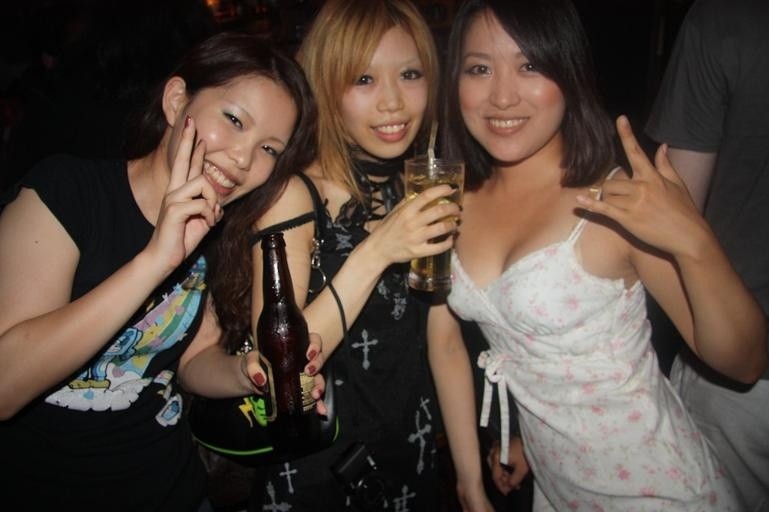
[0,32,315,510]
[253,1,462,512]
[645,1,768,510]
[427,0,768,511]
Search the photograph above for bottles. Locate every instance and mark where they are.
[257,230,319,433]
